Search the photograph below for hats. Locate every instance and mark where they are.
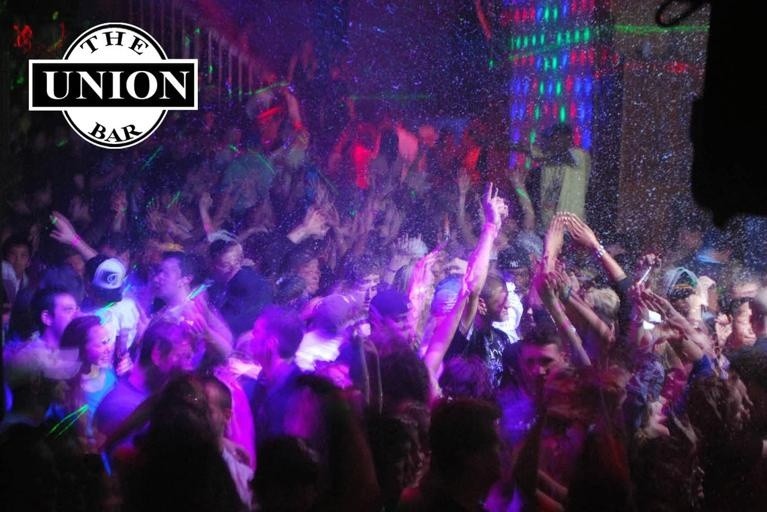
[86,255,123,301]
[663,267,698,297]
[369,291,414,316]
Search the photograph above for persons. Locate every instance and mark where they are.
[0,53,766,512]
